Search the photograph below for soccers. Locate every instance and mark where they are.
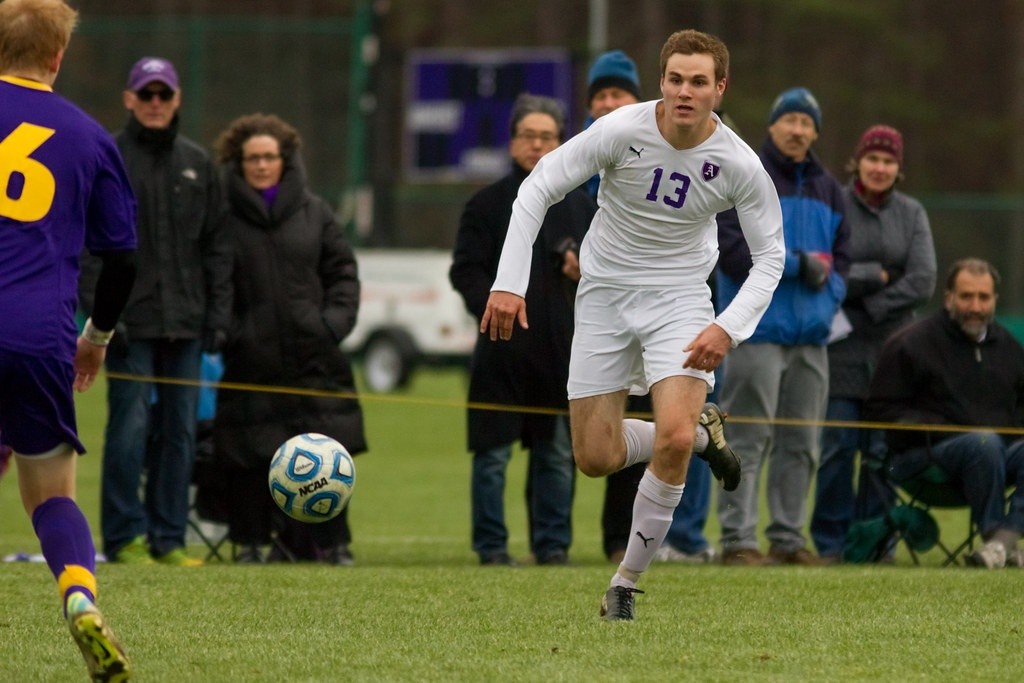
[268,432,356,524]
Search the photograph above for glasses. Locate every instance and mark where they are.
[137,88,173,102]
[243,152,282,161]
[515,133,558,145]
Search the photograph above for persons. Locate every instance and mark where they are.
[866,257,1023,568]
[651,380,720,564]
[195,112,368,568]
[449,89,598,570]
[810,123,939,567]
[478,29,787,623]
[559,49,655,568]
[713,85,858,567]
[77,57,234,571]
[0,0,141,683]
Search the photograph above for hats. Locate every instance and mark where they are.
[584,50,641,105]
[856,125,904,163]
[129,56,178,91]
[769,88,823,131]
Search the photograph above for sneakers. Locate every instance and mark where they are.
[600,587,645,623]
[159,548,202,570]
[721,548,772,567]
[962,542,1007,570]
[766,543,832,568]
[699,403,741,492]
[70,608,129,683]
[117,536,165,569]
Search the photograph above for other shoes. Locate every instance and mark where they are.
[535,547,569,567]
[480,553,518,567]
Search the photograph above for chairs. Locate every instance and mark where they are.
[837,397,1018,568]
[137,352,326,563]
